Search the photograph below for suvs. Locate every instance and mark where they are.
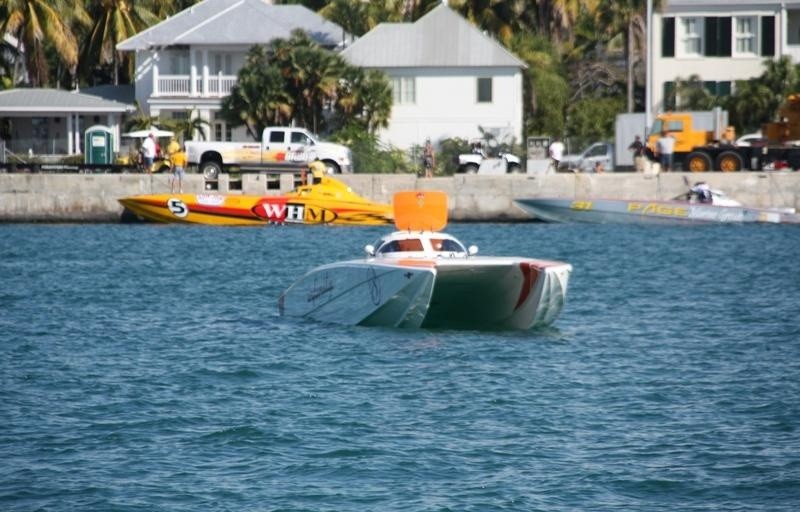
[457,151,522,174]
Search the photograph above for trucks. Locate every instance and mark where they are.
[644,113,799,170]
[557,109,729,173]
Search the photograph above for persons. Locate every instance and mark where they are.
[167,136,179,163]
[140,133,155,171]
[168,149,187,191]
[629,135,643,158]
[655,130,676,171]
[549,136,565,172]
[299,157,326,185]
[424,139,435,176]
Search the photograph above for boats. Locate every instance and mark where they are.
[673,177,742,208]
[116,175,394,225]
[278,191,574,329]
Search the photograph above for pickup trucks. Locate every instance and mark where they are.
[184,126,353,182]
